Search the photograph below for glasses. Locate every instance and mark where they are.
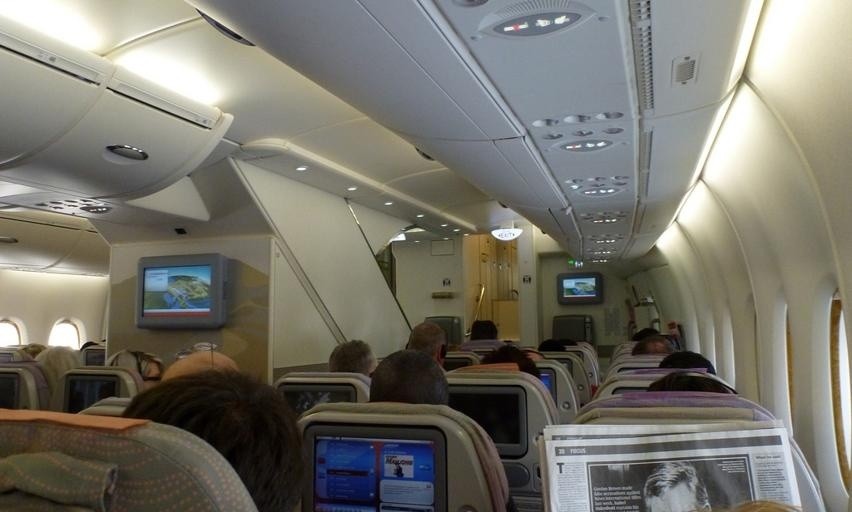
[491,221,523,241]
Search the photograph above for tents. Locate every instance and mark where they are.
[405,320,448,359]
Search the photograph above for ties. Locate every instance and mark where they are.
[0,372,19,409]
[446,385,528,459]
[0,353,13,364]
[64,374,118,415]
[557,272,603,305]
[84,350,105,366]
[279,384,356,422]
[444,344,661,404]
[134,254,228,329]
[303,425,447,511]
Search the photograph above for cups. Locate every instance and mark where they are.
[176,343,217,369]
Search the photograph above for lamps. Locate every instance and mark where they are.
[491,221,523,241]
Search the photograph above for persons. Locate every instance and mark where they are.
[406,322,449,375]
[393,459,405,477]
[558,337,578,348]
[538,338,565,351]
[370,349,451,408]
[34,347,83,394]
[471,319,498,341]
[659,350,716,376]
[642,461,714,512]
[631,335,674,356]
[481,344,541,381]
[119,364,310,512]
[328,339,378,377]
[632,327,659,342]
[647,371,723,393]
[106,348,164,394]
[161,351,242,381]
[22,343,46,359]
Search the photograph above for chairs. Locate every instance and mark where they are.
[0,338,825,512]
